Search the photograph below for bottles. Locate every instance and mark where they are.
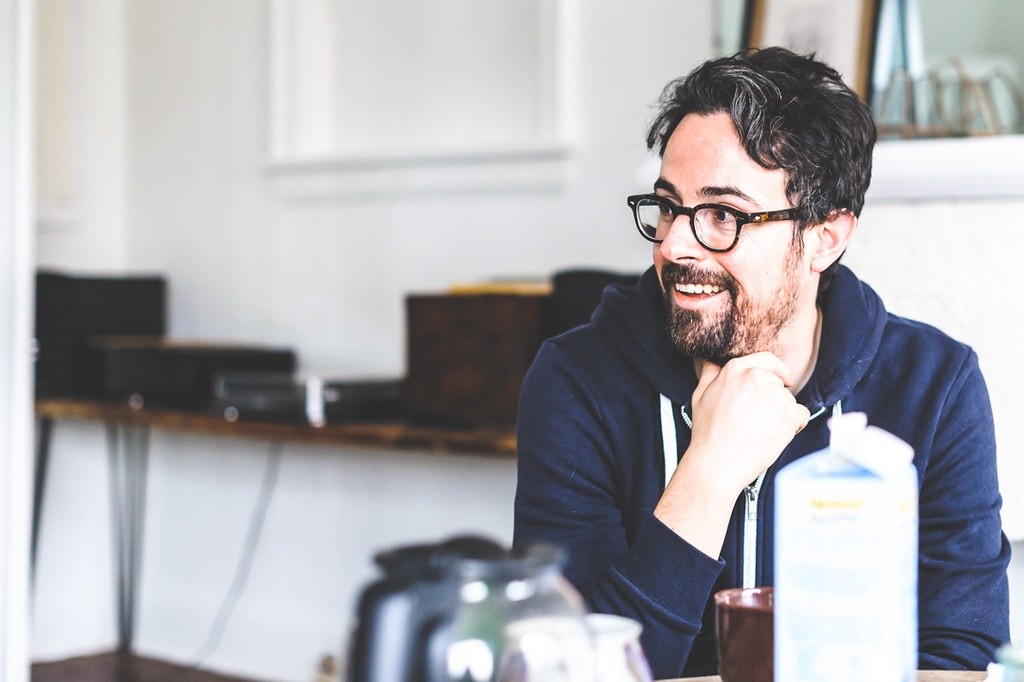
[712,586,774,680]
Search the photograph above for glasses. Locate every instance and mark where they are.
[626,189,810,252]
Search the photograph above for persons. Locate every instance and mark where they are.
[517,53,1009,680]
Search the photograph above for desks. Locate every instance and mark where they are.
[31,400,518,682]
